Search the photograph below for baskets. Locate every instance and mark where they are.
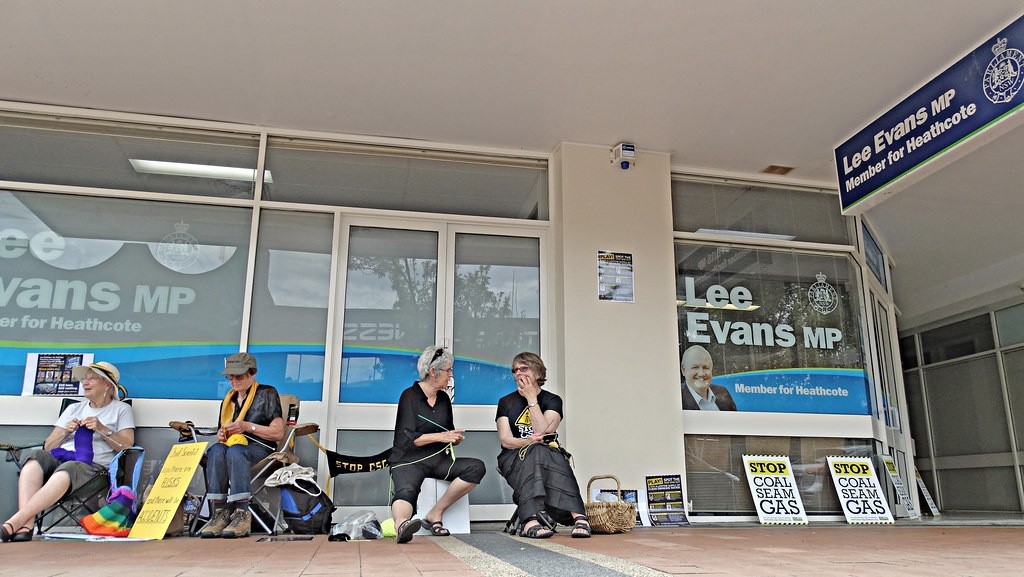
[585,475,636,534]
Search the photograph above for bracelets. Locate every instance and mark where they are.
[527,402,538,407]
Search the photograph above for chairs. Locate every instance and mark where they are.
[0,398,145,536]
[169,395,301,537]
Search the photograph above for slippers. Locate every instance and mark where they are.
[422,519,450,536]
[396,519,421,544]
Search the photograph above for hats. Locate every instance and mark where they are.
[222,353,256,375]
[72,361,120,401]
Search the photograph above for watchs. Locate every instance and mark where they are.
[101,430,113,439]
[251,423,256,434]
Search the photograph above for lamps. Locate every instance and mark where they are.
[693,228,797,241]
[129,158,274,185]
[676,300,761,311]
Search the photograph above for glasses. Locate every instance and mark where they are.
[431,349,444,364]
[80,376,100,383]
[226,374,244,380]
[512,367,529,374]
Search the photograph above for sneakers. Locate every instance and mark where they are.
[222,511,251,538]
[201,509,230,538]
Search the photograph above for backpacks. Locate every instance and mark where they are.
[281,480,336,534]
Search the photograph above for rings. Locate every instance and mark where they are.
[70,425,72,427]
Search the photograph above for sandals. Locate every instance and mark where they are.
[571,516,591,538]
[519,517,553,539]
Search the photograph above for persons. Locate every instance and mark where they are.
[200,352,286,539]
[681,345,737,412]
[388,346,486,544]
[0,361,135,542]
[494,352,590,539]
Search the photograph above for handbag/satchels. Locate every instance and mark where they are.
[264,463,322,496]
[79,446,146,537]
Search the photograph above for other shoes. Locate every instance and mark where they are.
[11,526,35,541]
[0,522,13,543]
